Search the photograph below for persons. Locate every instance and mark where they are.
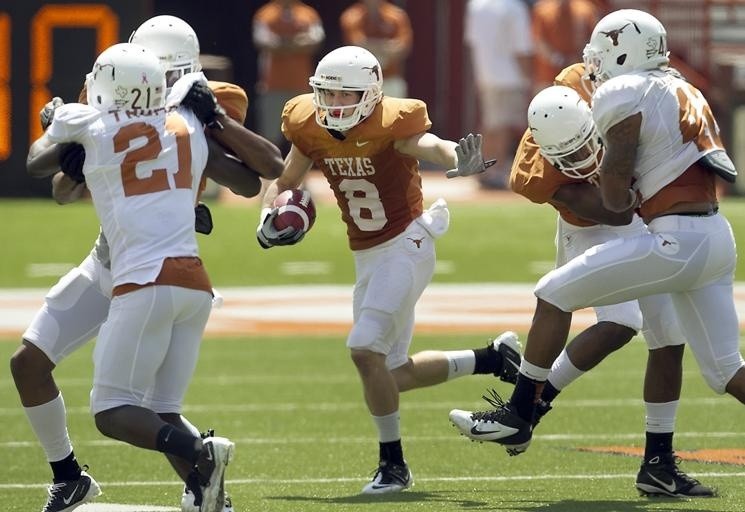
[450,9,745,457]
[460,1,536,193]
[532,1,602,92]
[338,1,414,99]
[27,43,262,512]
[508,61,718,498]
[9,15,284,512]
[256,46,523,494]
[250,0,325,147]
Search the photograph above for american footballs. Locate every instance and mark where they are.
[272,190,316,234]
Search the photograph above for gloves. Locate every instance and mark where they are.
[59,142,85,184]
[445,132,497,178]
[256,206,305,249]
[39,96,64,133]
[181,81,227,131]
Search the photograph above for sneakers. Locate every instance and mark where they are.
[486,330,522,385]
[181,429,235,512]
[635,451,719,498]
[361,459,413,494]
[41,464,102,512]
[449,386,552,456]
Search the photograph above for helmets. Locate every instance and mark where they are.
[527,85,606,178]
[580,9,670,98]
[86,42,167,111]
[309,45,384,132]
[128,14,202,100]
